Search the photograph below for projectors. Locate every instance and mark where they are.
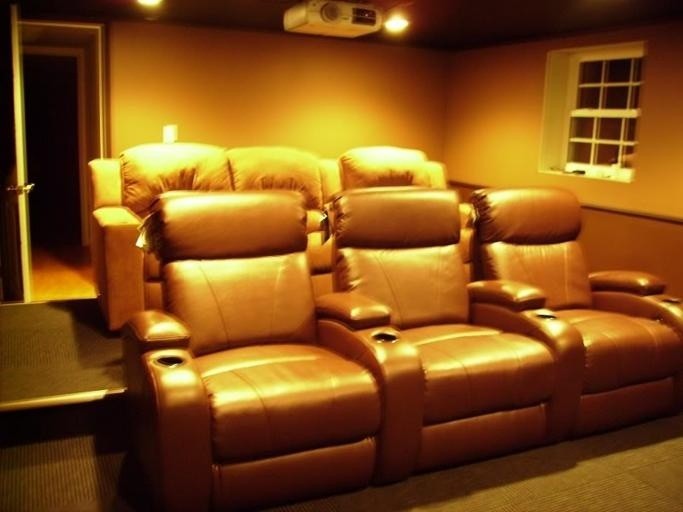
[283,0,382,39]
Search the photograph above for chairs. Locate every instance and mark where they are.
[122,185,683,512]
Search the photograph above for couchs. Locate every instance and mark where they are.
[85,146,477,337]
